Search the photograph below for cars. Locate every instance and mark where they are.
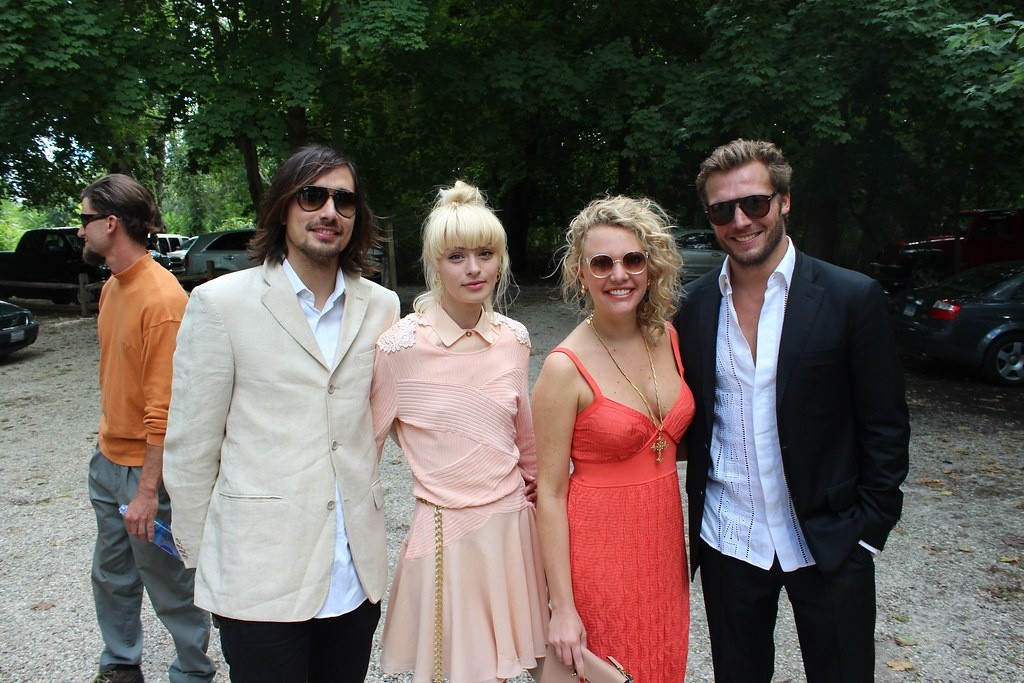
[669,228,727,286]
[13,226,104,305]
[168,227,265,287]
[872,206,1024,288]
[0,300,41,355]
[146,232,190,257]
[356,246,384,279]
[892,260,1024,384]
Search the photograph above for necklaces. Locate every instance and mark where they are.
[587,309,671,467]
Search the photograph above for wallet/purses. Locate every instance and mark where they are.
[525,641,633,683]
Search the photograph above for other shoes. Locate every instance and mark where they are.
[92,668,145,683]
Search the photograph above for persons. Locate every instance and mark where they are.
[532,194,696,683]
[76,172,219,683]
[161,145,538,681]
[654,137,913,683]
[365,178,553,680]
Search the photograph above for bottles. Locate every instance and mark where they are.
[118,504,184,563]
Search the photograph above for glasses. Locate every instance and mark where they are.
[580,250,648,278]
[80,213,108,228]
[295,185,361,218]
[704,189,779,226]
